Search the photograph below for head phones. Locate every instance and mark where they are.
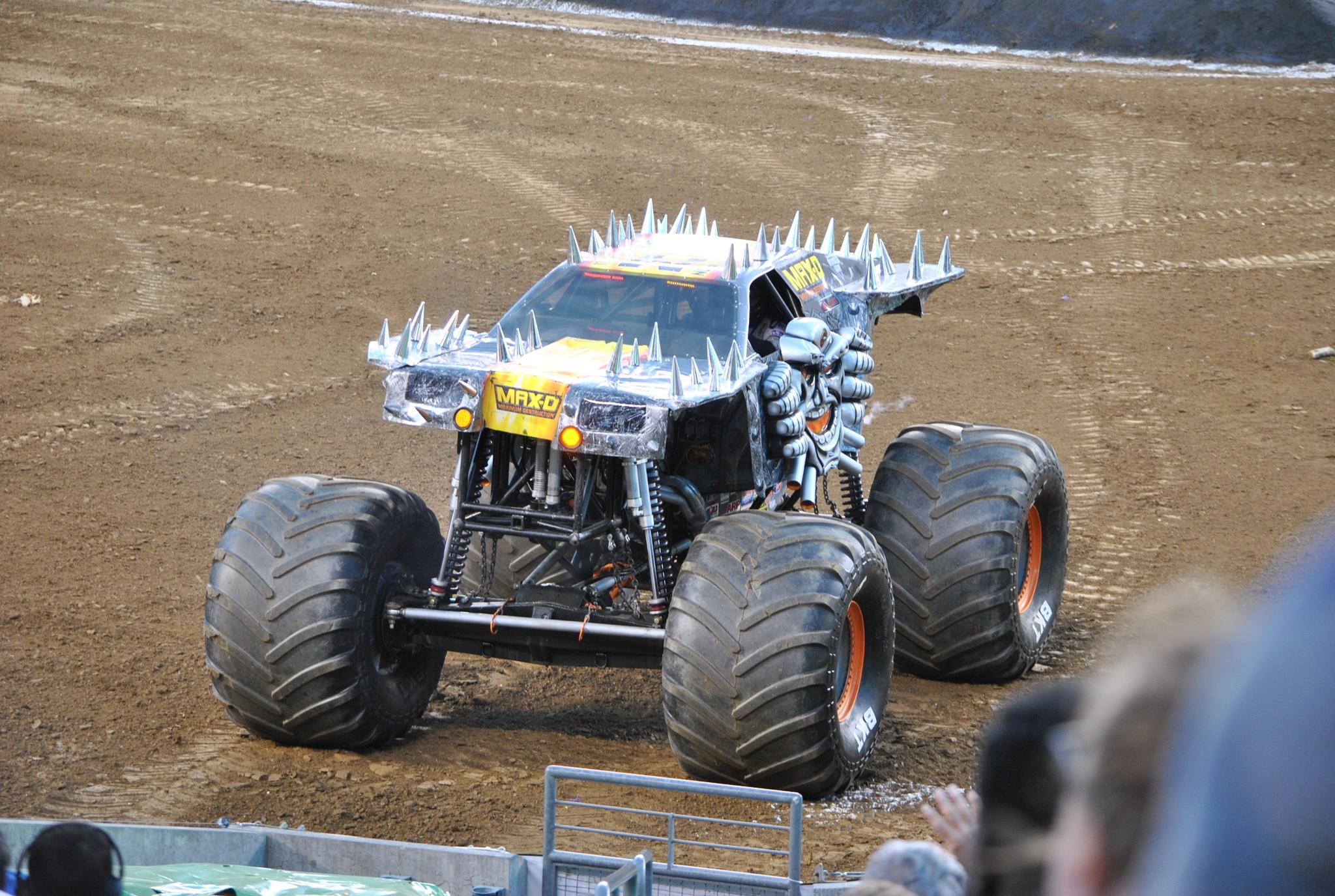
[15,822,124,896]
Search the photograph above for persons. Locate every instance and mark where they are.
[681,282,737,337]
[863,520,1335,895]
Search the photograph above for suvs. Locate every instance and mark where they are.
[201,196,1070,801]
